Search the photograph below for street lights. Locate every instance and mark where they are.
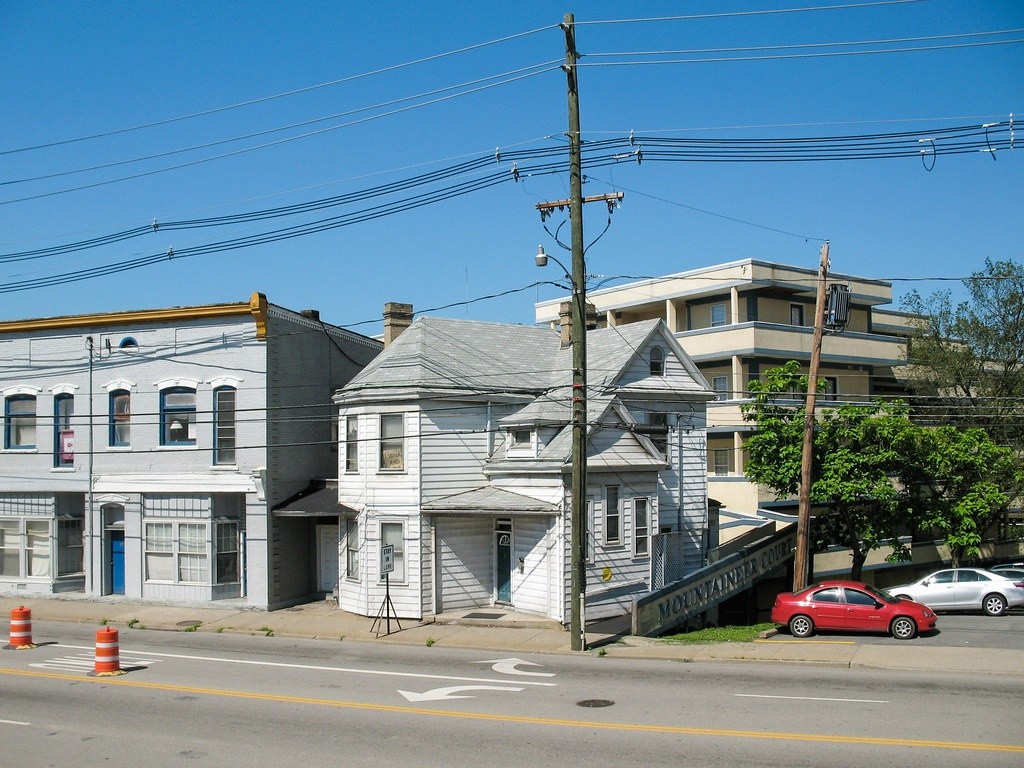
[535,244,589,652]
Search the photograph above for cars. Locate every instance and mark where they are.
[990,563,1024,581]
[875,567,1024,617]
[770,578,938,640]
